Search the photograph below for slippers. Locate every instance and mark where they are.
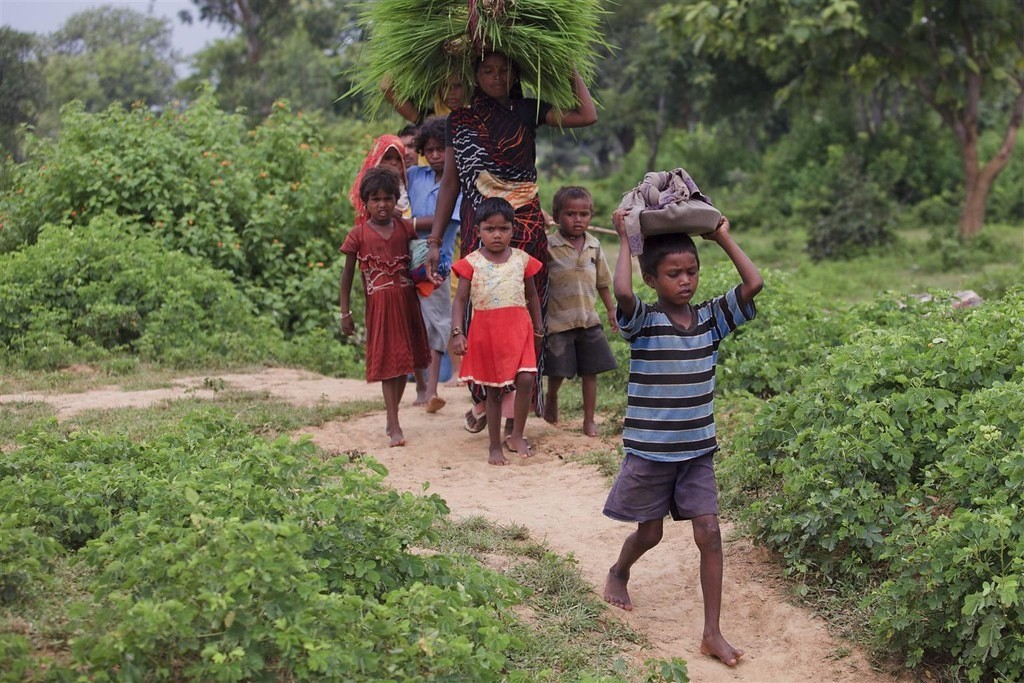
[504,435,531,452]
[465,407,487,433]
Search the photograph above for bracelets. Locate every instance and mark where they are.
[450,326,463,337]
[413,216,417,230]
[426,234,443,248]
[340,309,352,319]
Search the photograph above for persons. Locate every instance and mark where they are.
[349,60,555,412]
[543,186,618,438]
[339,167,434,446]
[450,196,544,467]
[424,50,599,436]
[603,203,763,667]
